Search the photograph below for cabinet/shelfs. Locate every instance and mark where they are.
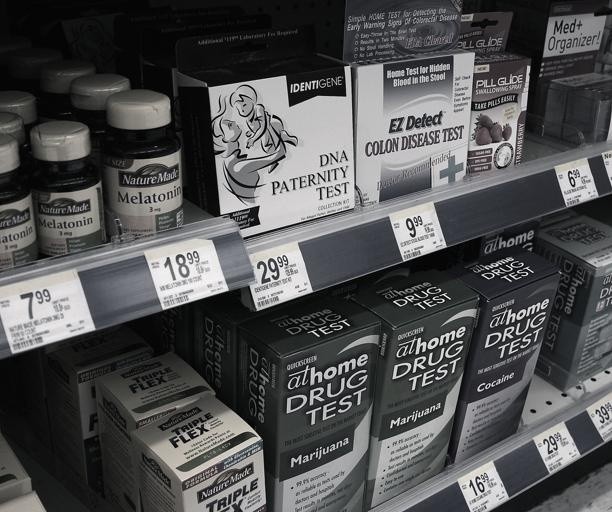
[0,135,612,512]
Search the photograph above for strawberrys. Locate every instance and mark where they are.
[475,115,511,145]
